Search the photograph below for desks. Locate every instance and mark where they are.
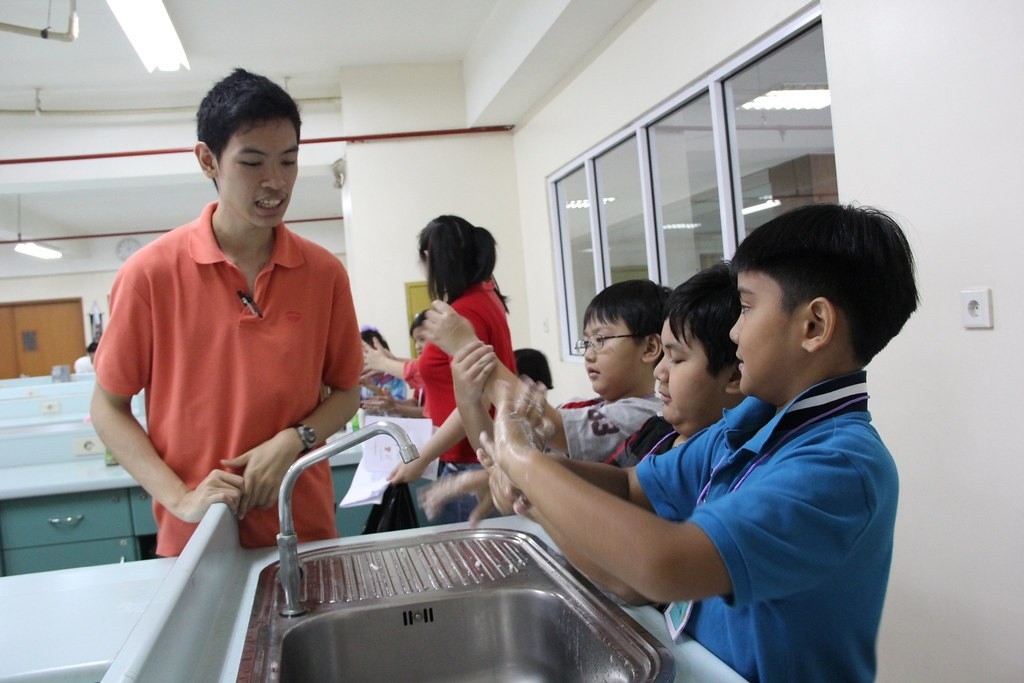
[0,430,459,581]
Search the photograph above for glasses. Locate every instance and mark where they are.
[574,332,645,357]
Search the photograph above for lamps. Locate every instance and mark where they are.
[331,159,346,189]
[14,193,63,261]
[106,0,191,76]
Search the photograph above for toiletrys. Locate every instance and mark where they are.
[345,408,365,434]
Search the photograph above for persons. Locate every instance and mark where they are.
[71,342,101,374]
[339,200,922,683]
[90,69,362,558]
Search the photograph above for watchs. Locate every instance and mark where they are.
[289,419,320,456]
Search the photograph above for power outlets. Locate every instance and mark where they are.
[959,289,995,329]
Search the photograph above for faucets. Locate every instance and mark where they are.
[275,420,420,618]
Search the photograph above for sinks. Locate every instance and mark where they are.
[235,583,678,683]
